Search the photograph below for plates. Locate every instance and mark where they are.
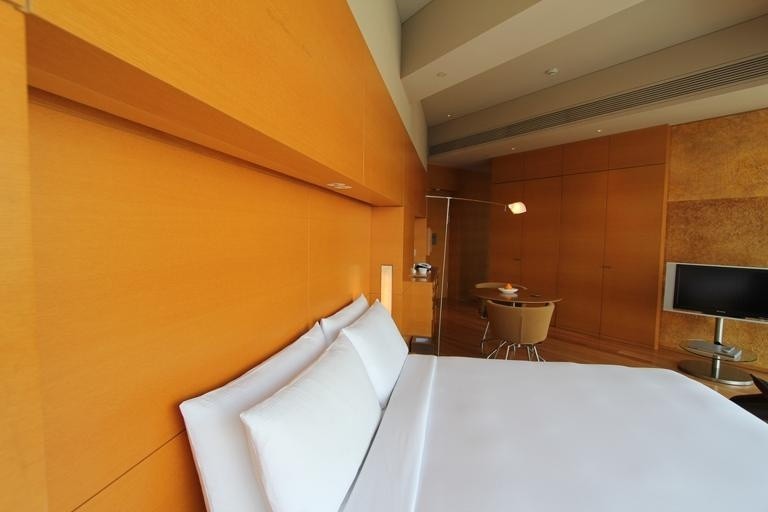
[498,287,519,294]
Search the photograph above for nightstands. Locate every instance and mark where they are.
[401,336,414,352]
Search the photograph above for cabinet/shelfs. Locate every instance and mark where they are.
[485,145,558,339]
[558,124,669,347]
[403,267,441,338]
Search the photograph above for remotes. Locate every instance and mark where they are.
[722,346,735,353]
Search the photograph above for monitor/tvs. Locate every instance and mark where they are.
[673,264,768,321]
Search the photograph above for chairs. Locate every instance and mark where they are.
[474,282,528,349]
[486,300,555,361]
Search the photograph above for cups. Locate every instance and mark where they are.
[418,268,427,275]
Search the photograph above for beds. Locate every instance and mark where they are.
[342,354,767,512]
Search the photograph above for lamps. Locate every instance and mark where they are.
[426,195,526,357]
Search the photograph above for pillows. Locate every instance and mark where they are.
[341,298,410,408]
[239,330,382,511]
[320,294,369,346]
[179,322,329,512]
